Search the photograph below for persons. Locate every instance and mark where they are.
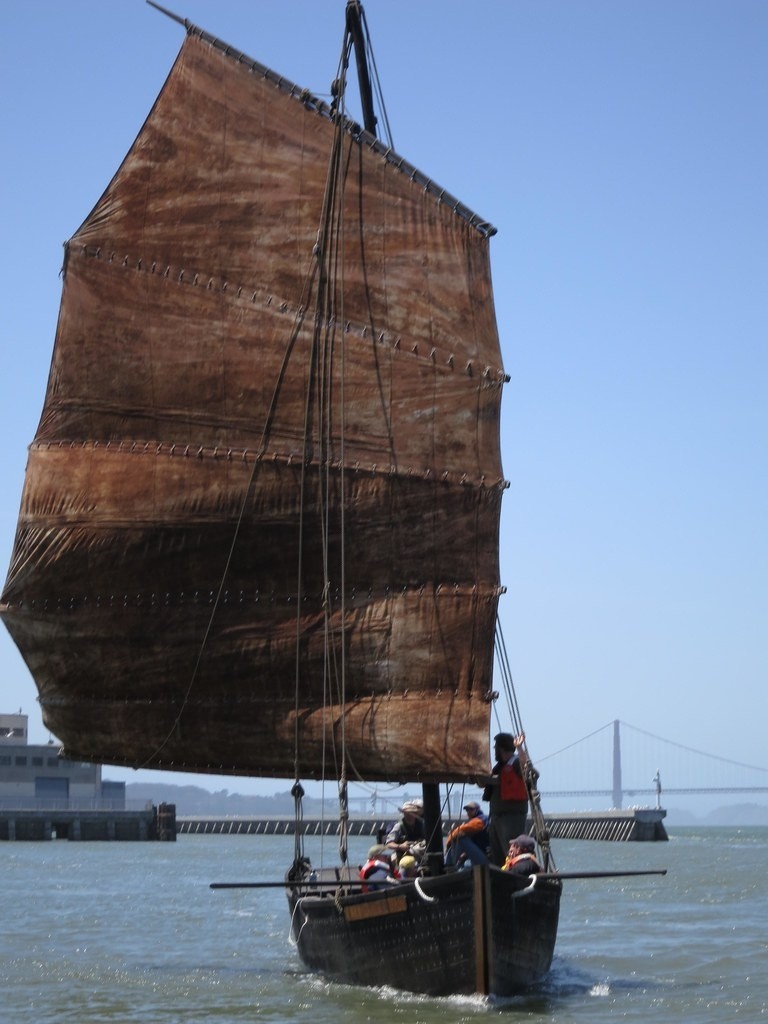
[384,798,425,865]
[359,844,401,893]
[445,801,489,866]
[399,856,415,880]
[483,732,540,866]
[502,835,542,877]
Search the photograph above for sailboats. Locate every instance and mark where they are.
[2,0,564,1003]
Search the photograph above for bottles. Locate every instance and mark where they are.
[309,869,317,889]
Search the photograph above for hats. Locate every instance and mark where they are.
[399,855,415,869]
[367,843,393,859]
[509,834,536,850]
[463,801,480,809]
